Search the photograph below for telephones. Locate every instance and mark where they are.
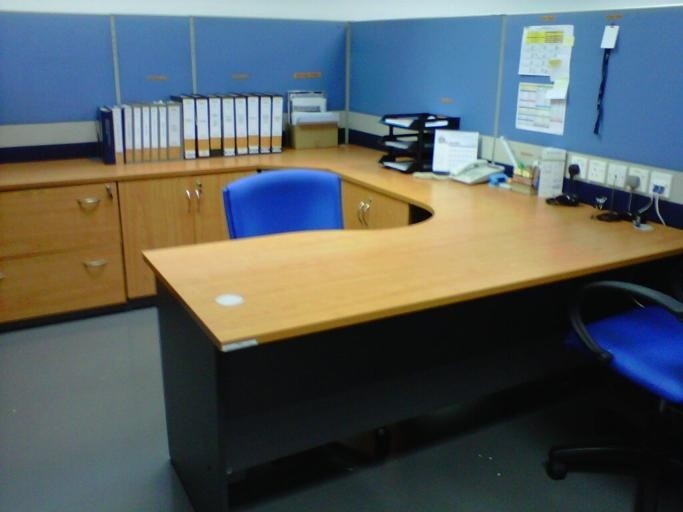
[448,158,505,185]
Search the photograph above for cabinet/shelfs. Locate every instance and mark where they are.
[0,139,683,512]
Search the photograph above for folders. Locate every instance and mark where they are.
[97,90,284,165]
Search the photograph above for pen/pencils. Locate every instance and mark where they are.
[500,136,519,167]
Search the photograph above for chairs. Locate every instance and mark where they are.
[223,167,345,241]
[544,281,683,512]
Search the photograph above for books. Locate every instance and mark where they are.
[380,110,447,174]
[284,88,340,126]
[94,86,282,166]
